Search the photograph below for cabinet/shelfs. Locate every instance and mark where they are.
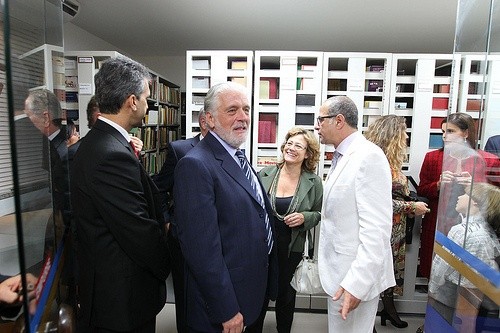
[61,50,182,179]
[185,49,500,313]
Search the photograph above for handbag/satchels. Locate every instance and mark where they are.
[290,230,327,295]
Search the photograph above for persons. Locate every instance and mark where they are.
[364,114,431,333]
[0,56,70,321]
[314,96,397,333]
[69,59,171,332]
[418,112,500,333]
[159,80,278,333]
[244,127,324,333]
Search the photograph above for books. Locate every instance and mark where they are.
[131,82,180,176]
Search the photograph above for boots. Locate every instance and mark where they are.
[323,151,340,182]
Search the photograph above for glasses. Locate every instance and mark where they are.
[317,115,337,122]
[72,131,80,136]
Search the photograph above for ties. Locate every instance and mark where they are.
[235,149,275,256]
[130,141,139,158]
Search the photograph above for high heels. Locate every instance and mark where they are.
[381,308,408,328]
